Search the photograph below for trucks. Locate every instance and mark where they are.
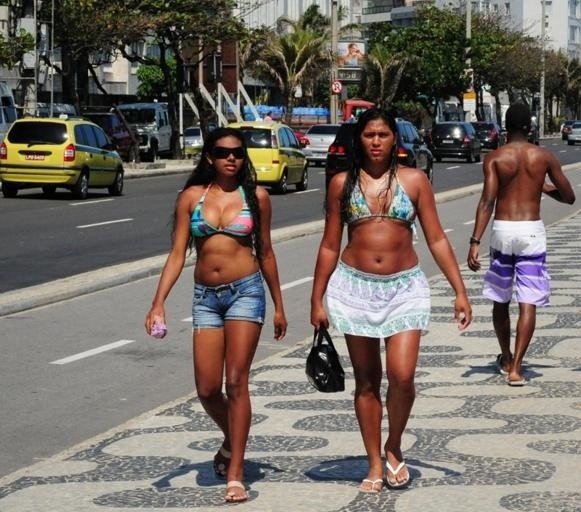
[117,102,171,160]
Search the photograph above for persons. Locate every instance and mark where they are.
[144,127,288,504]
[264,111,272,121]
[309,110,471,493]
[467,103,576,386]
[339,43,363,67]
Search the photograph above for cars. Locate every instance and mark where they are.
[0,117,124,198]
[567,122,581,145]
[528,124,539,145]
[303,124,340,165]
[83,112,138,162]
[225,121,308,194]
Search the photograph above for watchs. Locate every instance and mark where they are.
[469,237,481,245]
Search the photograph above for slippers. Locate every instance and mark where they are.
[212,445,233,478]
[384,459,411,489]
[496,353,510,375]
[222,480,248,502]
[505,375,526,386]
[358,477,385,494]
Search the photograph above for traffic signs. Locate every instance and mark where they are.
[332,81,342,94]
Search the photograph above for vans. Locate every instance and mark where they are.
[184,126,214,146]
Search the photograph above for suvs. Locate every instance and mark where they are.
[472,121,505,151]
[431,122,481,163]
[325,117,434,190]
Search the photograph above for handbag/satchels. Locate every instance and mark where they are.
[305,326,345,394]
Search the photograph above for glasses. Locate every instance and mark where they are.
[210,146,246,160]
[520,125,531,132]
